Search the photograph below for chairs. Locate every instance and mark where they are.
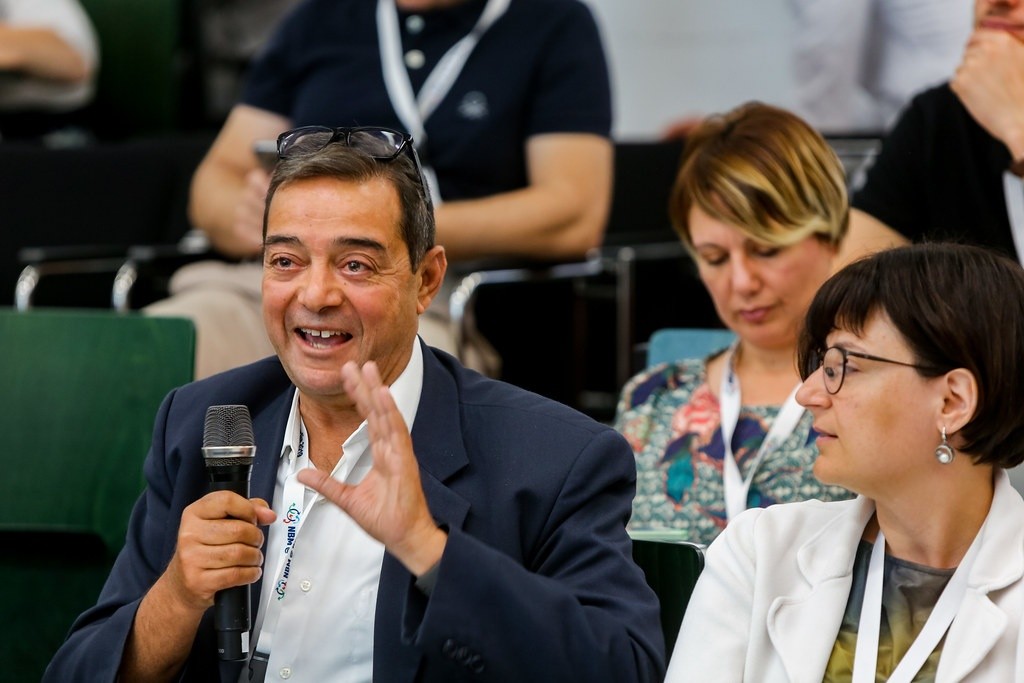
[0,0,707,683]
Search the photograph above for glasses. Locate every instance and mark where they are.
[804,343,944,394]
[276,124,433,217]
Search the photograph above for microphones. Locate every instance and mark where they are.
[202,404,256,661]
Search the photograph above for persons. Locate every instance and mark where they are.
[662,243,1024,683]
[0,0,104,112]
[834,0,1024,270]
[141,0,614,382]
[35,126,663,682]
[617,105,857,548]
